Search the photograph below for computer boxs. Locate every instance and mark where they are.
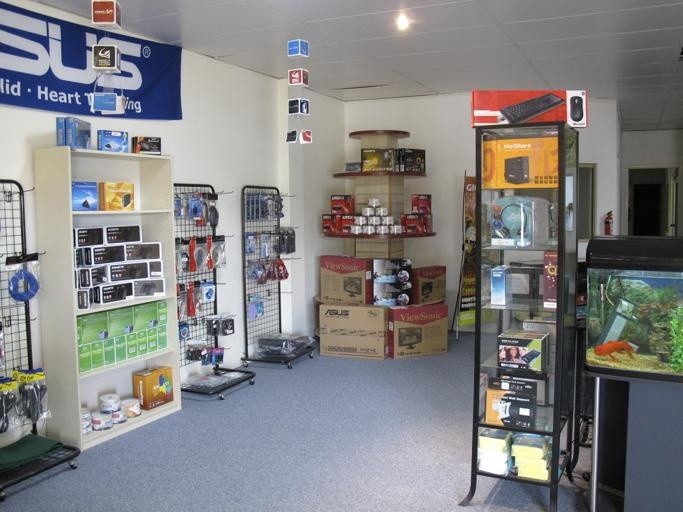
[505,156,529,184]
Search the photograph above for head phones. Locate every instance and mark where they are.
[500,203,528,238]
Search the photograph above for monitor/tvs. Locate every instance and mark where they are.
[399,327,422,349]
[344,276,361,298]
[421,281,433,298]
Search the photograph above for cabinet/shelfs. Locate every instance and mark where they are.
[459,121,586,512]
[31,145,183,452]
[313,129,438,339]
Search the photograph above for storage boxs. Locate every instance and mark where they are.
[318,255,450,361]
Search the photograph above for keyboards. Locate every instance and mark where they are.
[500,93,565,123]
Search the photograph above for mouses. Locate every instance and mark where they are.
[569,95,584,122]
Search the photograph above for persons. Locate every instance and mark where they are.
[498,344,527,364]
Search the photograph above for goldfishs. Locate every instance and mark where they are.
[594,339,639,362]
